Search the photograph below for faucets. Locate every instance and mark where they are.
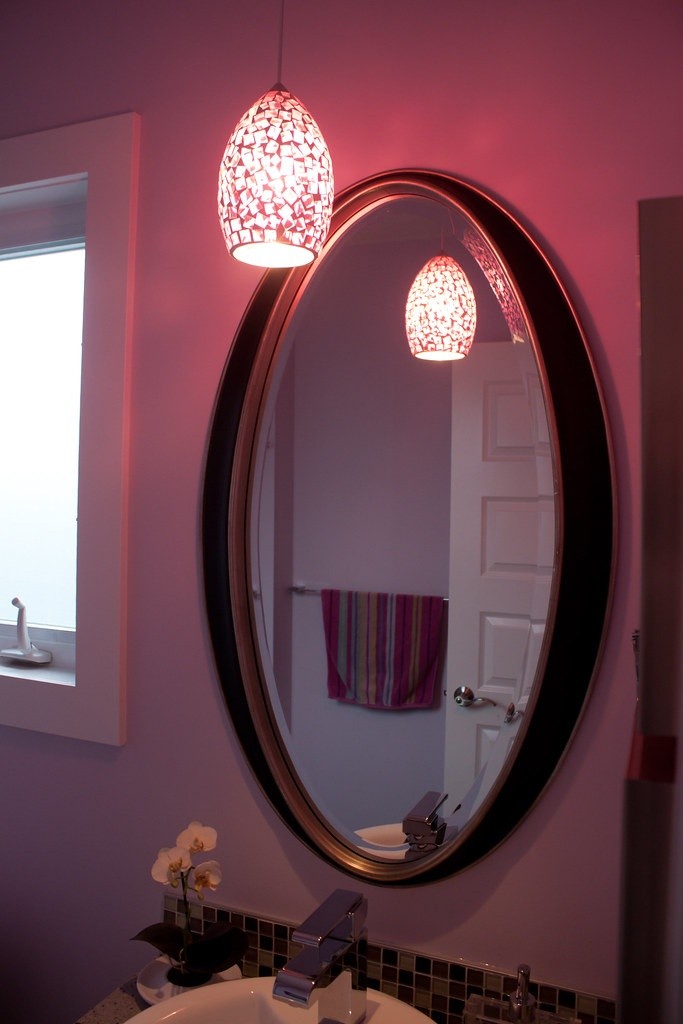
[397,785,449,849]
[271,886,379,1024]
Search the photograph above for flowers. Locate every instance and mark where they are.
[126,818,250,981]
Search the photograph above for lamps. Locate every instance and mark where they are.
[214,0,335,268]
[405,204,477,361]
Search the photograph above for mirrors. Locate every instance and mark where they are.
[226,178,566,887]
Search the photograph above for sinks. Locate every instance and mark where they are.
[114,975,443,1024]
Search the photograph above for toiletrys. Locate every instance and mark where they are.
[461,963,582,1024]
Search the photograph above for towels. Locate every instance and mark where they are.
[318,586,449,713]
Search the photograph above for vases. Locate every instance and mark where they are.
[135,949,244,1007]
